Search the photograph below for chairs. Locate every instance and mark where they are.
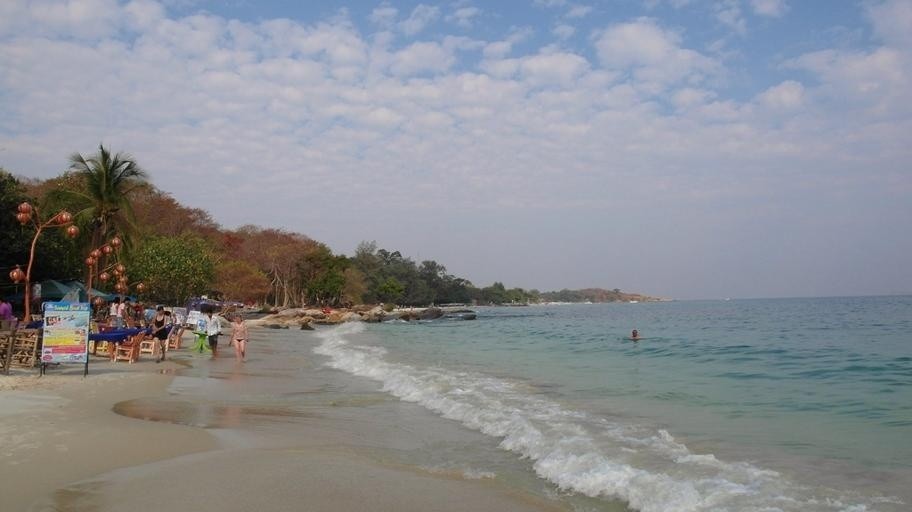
[0,318,209,375]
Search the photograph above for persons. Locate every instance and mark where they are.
[0,297,14,320]
[623,329,641,342]
[397,305,399,311]
[110,297,249,363]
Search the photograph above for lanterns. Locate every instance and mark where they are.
[10,202,146,310]
